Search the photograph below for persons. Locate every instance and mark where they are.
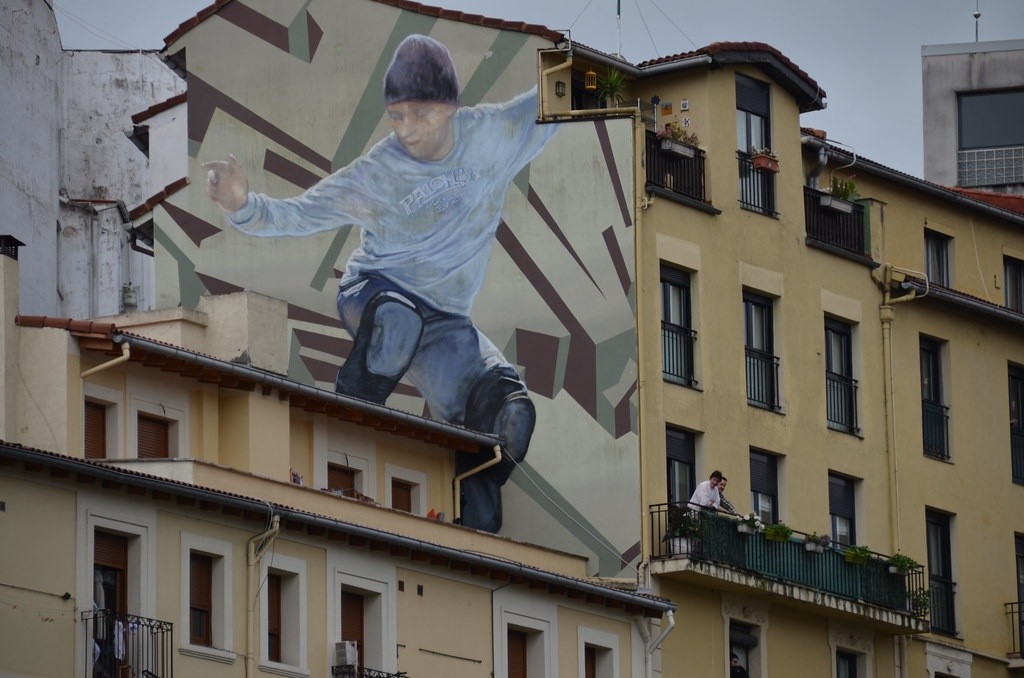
[717,476,744,518]
[729,651,749,678]
[686,469,722,516]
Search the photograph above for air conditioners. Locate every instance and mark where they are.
[335,641,358,666]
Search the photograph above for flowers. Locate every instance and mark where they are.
[743,510,765,534]
[655,114,701,150]
[751,145,776,159]
[831,174,860,201]
[806,531,832,548]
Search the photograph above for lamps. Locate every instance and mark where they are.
[555,81,565,97]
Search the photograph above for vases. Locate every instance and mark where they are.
[661,137,695,160]
[820,195,854,214]
[805,542,824,555]
[749,155,780,173]
[734,518,753,537]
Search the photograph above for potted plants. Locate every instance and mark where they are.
[843,546,872,565]
[661,505,699,558]
[593,65,626,116]
[765,520,792,543]
[889,553,920,576]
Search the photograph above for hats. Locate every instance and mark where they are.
[710,471,722,482]
